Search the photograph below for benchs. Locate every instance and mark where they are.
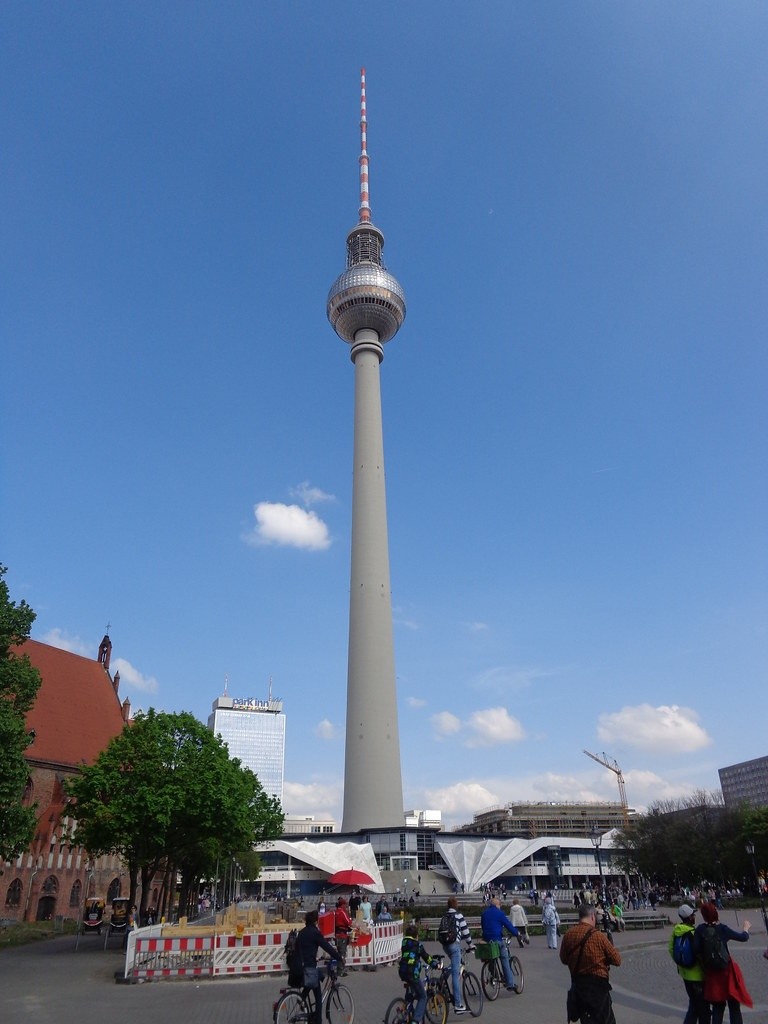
[419,911,669,942]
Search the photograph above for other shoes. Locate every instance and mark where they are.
[508,984,518,991]
[340,973,347,977]
[454,1006,471,1014]
[520,941,523,948]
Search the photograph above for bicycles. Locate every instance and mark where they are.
[423,946,484,1024]
[272,955,355,1024]
[381,963,449,1024]
[474,934,525,1002]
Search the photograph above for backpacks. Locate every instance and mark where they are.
[438,913,461,944]
[698,923,730,972]
[673,931,698,969]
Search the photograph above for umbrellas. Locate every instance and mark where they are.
[327,867,375,885]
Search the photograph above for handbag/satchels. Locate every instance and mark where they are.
[567,989,580,1024]
[303,967,319,988]
[524,925,529,944]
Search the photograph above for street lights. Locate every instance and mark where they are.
[744,838,763,904]
[672,860,678,884]
[588,825,605,888]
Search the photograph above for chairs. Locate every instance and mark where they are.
[85,907,103,932]
[110,914,128,932]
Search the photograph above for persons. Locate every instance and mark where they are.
[335,898,352,977]
[432,882,436,894]
[318,875,421,923]
[541,898,561,950]
[694,903,752,1024]
[201,891,305,912]
[296,910,345,1024]
[144,900,157,927]
[398,926,437,1024]
[481,900,520,992]
[559,904,621,1024]
[510,900,530,947]
[480,882,508,903]
[515,882,552,905]
[668,905,712,1024]
[443,897,477,1014]
[122,905,140,955]
[454,882,464,894]
[574,881,740,932]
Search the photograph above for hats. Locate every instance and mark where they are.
[338,899,346,906]
[678,905,698,918]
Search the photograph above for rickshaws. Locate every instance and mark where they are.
[74,897,107,951]
[104,897,131,951]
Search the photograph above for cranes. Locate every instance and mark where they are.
[583,748,630,830]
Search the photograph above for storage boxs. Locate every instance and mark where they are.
[475,941,500,960]
